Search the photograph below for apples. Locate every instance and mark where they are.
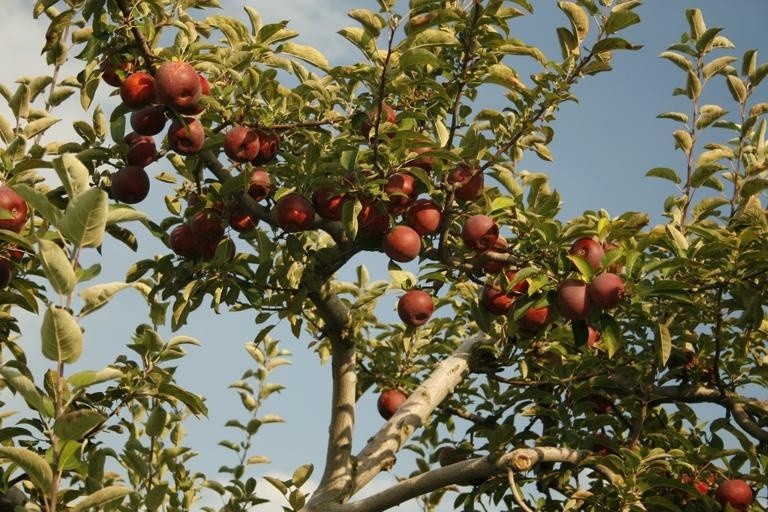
[0,185,28,290]
[668,348,697,370]
[101,58,626,349]
[592,434,608,456]
[715,479,753,512]
[592,393,616,413]
[377,390,408,421]
